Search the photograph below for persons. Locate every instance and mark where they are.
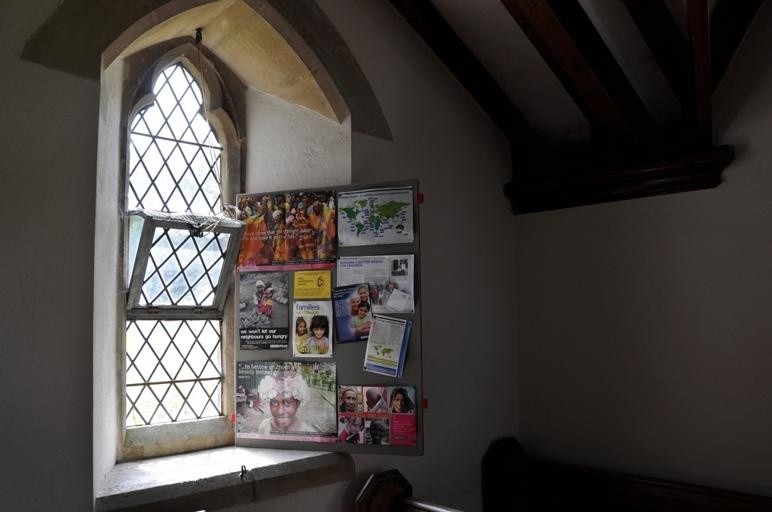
[295,311,329,355]
[257,366,317,433]
[236,188,336,268]
[251,278,277,317]
[338,387,415,447]
[349,285,371,340]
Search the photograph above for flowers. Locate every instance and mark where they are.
[257,374,311,406]
